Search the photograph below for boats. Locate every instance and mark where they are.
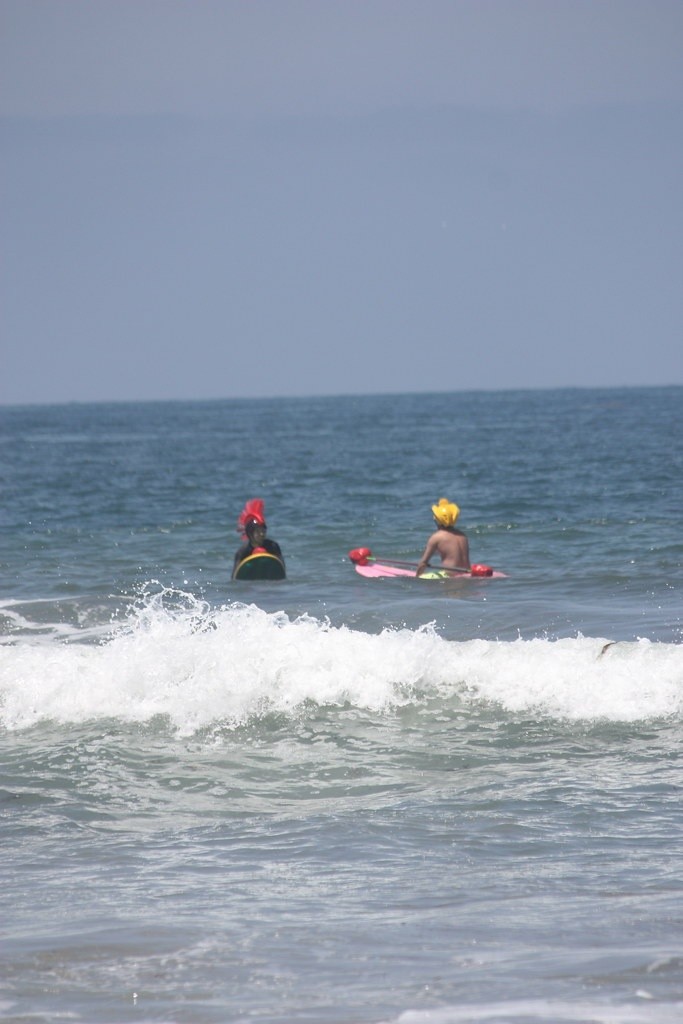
[234,552,286,580]
[354,563,506,579]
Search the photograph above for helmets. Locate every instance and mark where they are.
[237,499,266,540]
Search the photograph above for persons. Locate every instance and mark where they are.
[416,498,470,578]
[234,498,285,570]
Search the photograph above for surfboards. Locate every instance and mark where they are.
[233,552,286,581]
[355,563,505,580]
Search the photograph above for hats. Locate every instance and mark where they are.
[431,498,460,527]
